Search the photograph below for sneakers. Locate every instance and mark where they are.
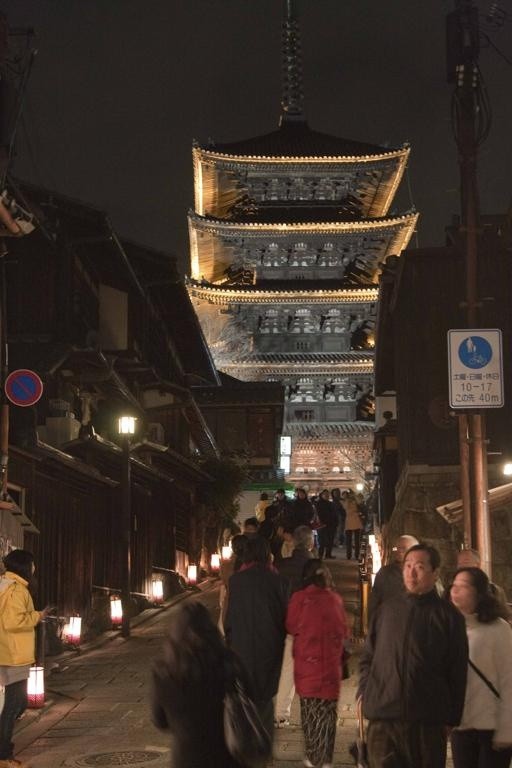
[319,554,337,560]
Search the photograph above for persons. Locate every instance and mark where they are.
[224,534,289,742]
[443,566,511,768]
[355,543,469,768]
[284,558,349,768]
[1,550,54,768]
[368,534,446,629]
[218,486,368,639]
[272,524,321,727]
[149,601,269,768]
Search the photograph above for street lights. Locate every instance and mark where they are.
[115,410,141,639]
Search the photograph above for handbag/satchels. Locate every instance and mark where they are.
[223,689,273,768]
[341,647,352,679]
